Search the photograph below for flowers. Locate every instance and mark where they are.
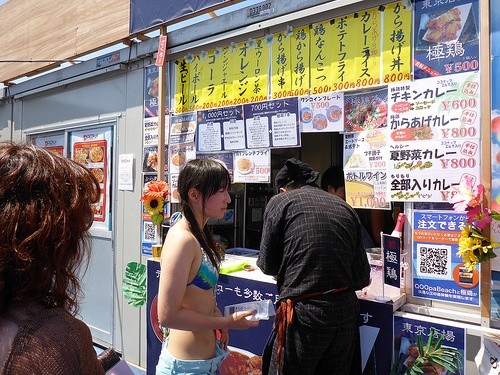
[449,175,500,273]
[140,180,171,228]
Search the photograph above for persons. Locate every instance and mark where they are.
[321,163,396,248]
[256,158,372,375]
[156,157,259,375]
[0,141,106,375]
